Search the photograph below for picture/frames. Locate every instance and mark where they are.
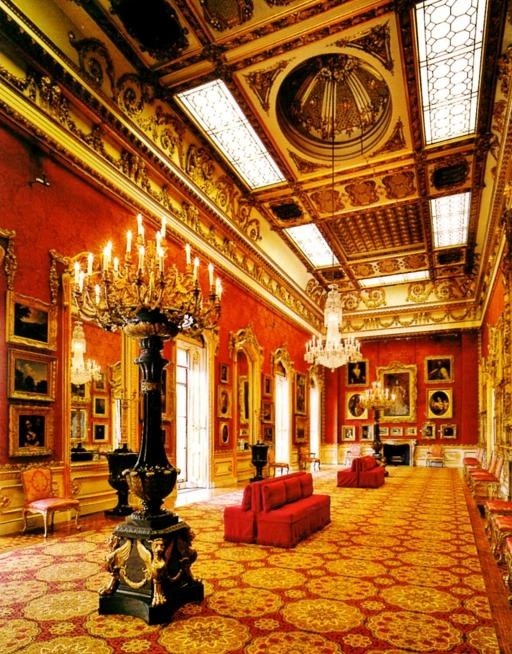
[6,344,59,404]
[72,359,112,449]
[7,399,57,458]
[213,337,456,449]
[5,286,62,351]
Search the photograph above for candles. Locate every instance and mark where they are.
[61,203,223,306]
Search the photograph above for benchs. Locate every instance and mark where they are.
[337,458,360,488]
[223,476,262,545]
[255,469,333,548]
[359,455,385,487]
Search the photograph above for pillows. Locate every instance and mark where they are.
[262,470,317,509]
[240,485,251,512]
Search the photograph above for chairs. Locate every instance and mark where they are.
[344,444,362,466]
[266,453,294,479]
[17,461,84,541]
[424,443,447,470]
[293,444,321,472]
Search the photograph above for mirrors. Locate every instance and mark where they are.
[59,268,132,469]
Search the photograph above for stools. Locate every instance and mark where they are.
[462,449,512,614]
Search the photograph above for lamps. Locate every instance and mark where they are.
[71,312,105,392]
[301,57,363,375]
[359,379,398,477]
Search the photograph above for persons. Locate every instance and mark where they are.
[389,379,409,415]
